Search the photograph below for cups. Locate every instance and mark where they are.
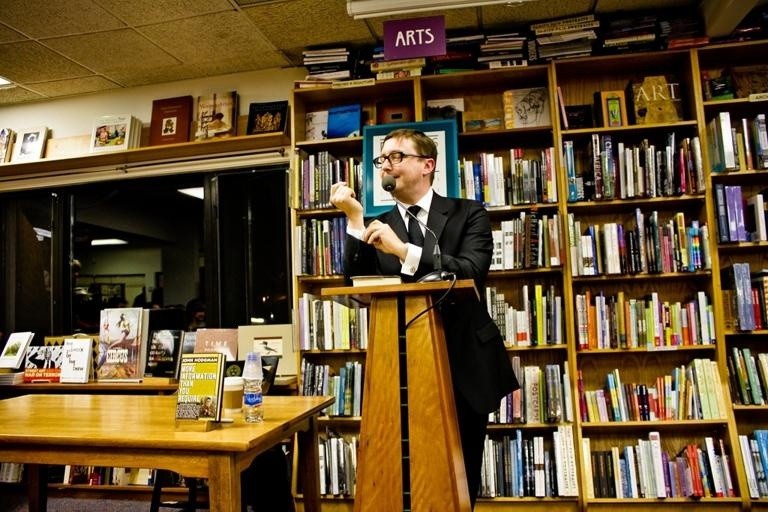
[222,376,245,415]
[241,351,263,380]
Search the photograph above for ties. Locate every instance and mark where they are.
[405,205,426,249]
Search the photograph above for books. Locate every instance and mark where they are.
[0,91,287,165]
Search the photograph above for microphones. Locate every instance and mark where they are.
[382,176,450,283]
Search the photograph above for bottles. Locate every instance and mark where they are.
[242,353,264,423]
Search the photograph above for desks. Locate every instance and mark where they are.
[1,392,337,512]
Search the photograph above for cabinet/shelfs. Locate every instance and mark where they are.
[550,47,745,510]
[1,380,297,499]
[289,63,580,510]
[693,36,767,507]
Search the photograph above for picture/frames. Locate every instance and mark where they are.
[11,124,49,163]
[360,118,459,220]
[237,322,297,377]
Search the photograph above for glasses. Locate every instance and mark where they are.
[371,151,428,169]
